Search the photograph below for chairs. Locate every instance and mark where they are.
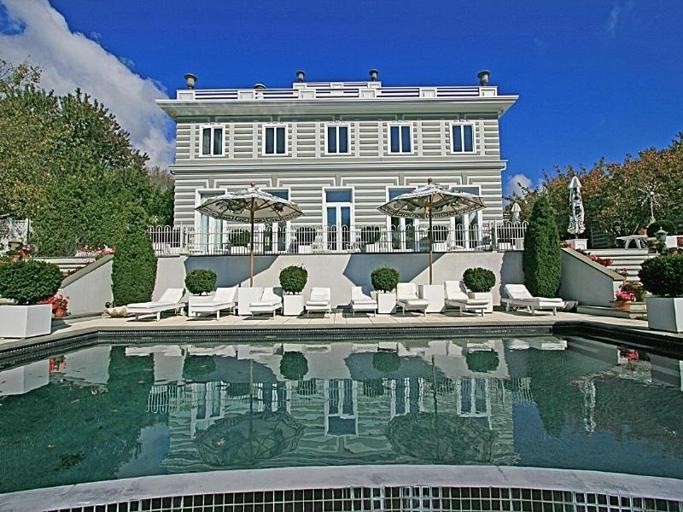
[444,278,489,318]
[248,284,283,321]
[393,279,429,319]
[500,281,565,318]
[305,284,332,319]
[189,286,238,321]
[124,287,186,322]
[349,284,377,319]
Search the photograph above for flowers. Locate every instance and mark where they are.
[615,278,647,304]
[46,295,69,315]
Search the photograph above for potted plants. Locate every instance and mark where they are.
[227,229,250,255]
[182,350,215,378]
[465,343,500,374]
[0,253,64,342]
[463,265,495,314]
[373,342,401,373]
[280,344,308,379]
[370,265,399,315]
[634,252,682,334]
[294,226,315,253]
[427,225,453,252]
[360,225,381,253]
[184,269,217,317]
[277,265,308,317]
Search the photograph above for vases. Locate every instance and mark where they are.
[616,300,628,310]
[55,309,64,317]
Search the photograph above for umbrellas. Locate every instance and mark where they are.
[581,377,596,438]
[382,349,500,465]
[509,201,521,224]
[192,182,304,286]
[565,174,586,238]
[194,354,306,468]
[376,176,483,282]
